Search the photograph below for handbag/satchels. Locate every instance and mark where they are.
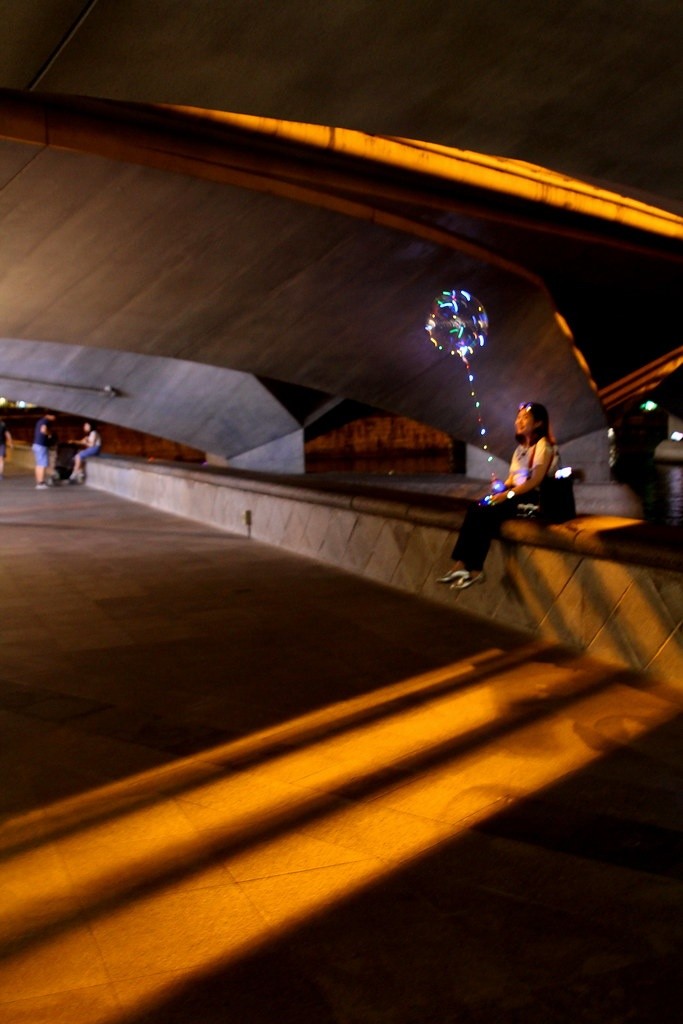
[516,476,576,524]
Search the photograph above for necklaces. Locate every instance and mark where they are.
[518,442,532,460]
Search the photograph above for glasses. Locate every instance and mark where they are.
[519,401,535,413]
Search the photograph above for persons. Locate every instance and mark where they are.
[434,399,562,590]
[53,430,82,485]
[61,421,103,485]
[30,409,57,490]
[0,421,15,480]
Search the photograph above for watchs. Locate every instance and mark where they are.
[506,488,516,499]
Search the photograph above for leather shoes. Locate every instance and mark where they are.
[436,569,486,590]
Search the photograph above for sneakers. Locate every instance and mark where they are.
[35,481,51,489]
[69,472,85,479]
